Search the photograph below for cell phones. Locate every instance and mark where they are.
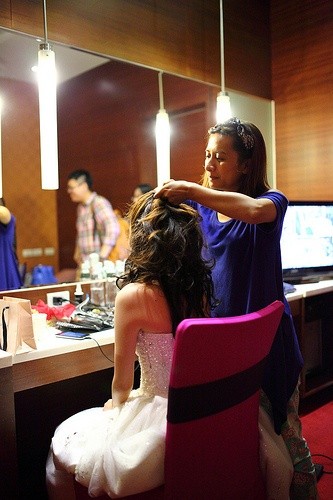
[56,331,89,340]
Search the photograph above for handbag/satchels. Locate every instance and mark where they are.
[0,296,37,356]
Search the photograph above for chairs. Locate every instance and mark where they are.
[133,300,287,500]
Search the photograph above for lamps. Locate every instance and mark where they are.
[155,72,171,184]
[214,0,231,122]
[36,1,59,190]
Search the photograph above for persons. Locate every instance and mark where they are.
[131,185,150,204]
[0,205,23,291]
[146,117,320,500]
[67,169,121,281]
[44,187,224,500]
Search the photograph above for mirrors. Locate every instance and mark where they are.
[1,28,273,293]
